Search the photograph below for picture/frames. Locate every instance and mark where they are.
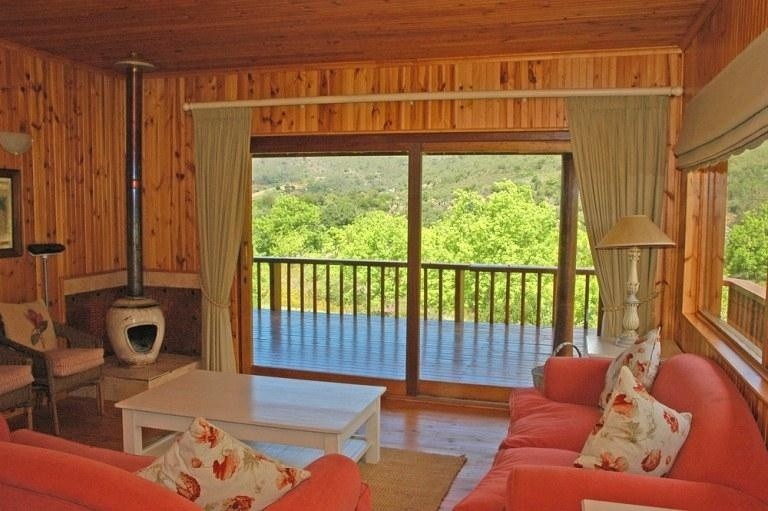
[0,169,24,258]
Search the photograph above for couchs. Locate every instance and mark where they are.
[0,414,372,511]
[454,352,768,510]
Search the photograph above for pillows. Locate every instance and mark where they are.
[573,366,692,478]
[598,327,662,409]
[135,417,311,511]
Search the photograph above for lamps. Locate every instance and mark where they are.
[596,214,676,347]
[0,132,32,154]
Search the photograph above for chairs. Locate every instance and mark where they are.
[0,346,34,430]
[0,299,106,435]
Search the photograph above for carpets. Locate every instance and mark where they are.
[356,446,467,510]
